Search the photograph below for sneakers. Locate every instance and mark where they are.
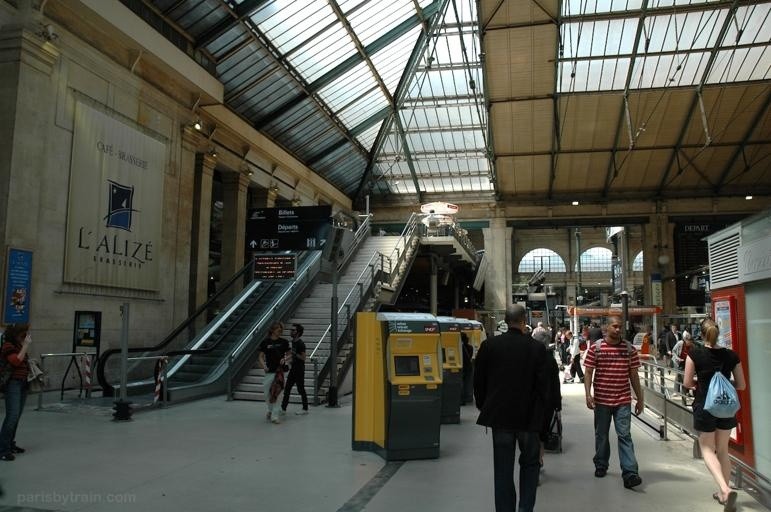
[594,466,606,478]
[0,443,27,461]
[624,472,642,487]
[264,408,310,424]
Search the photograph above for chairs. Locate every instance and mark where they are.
[426,218,439,237]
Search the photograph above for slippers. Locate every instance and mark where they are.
[713,489,739,512]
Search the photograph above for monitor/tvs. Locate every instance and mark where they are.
[442,348,446,363]
[394,356,420,376]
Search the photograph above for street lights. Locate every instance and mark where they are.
[619,289,629,337]
[576,296,584,333]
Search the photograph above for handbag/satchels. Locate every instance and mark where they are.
[545,430,564,452]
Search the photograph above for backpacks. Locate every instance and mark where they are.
[703,351,742,420]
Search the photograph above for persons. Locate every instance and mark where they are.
[682,318,745,512]
[460,318,707,406]
[257,319,309,424]
[0,321,33,461]
[473,305,561,512]
[582,316,645,489]
[426,209,440,227]
